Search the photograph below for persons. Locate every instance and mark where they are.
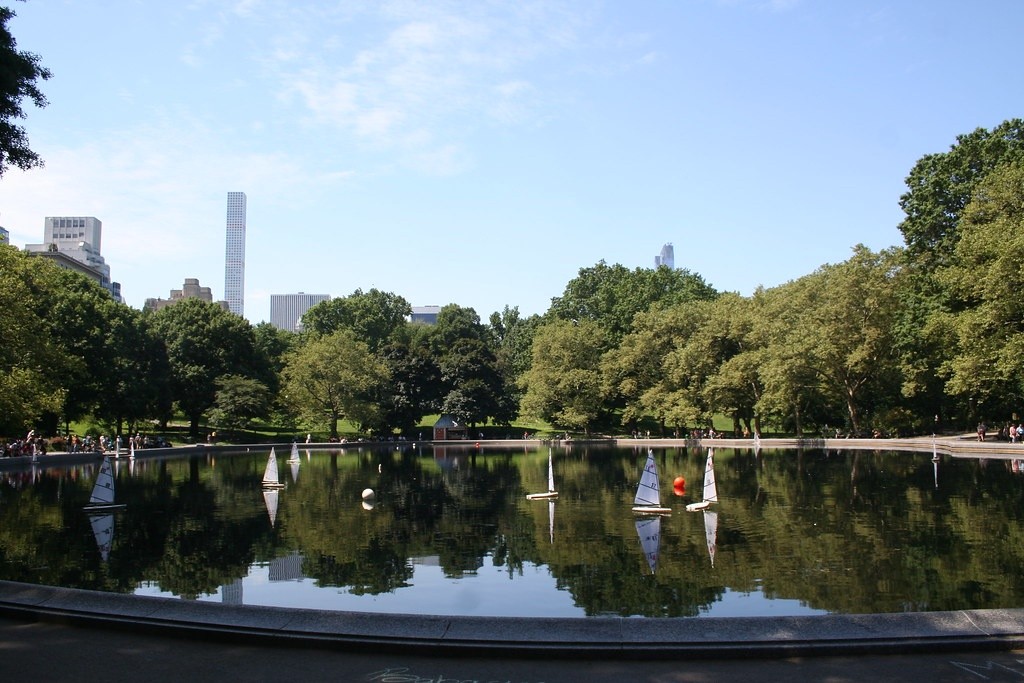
[0,425,217,457]
[632,429,651,439]
[524,431,572,440]
[978,419,1024,444]
[978,459,1024,473]
[479,430,483,440]
[693,427,751,438]
[823,424,889,439]
[305,431,407,442]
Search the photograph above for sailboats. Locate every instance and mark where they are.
[686,508,720,568]
[261,488,284,530]
[631,512,672,575]
[102,439,128,455]
[129,441,136,459]
[526,497,559,544]
[287,462,302,485]
[31,444,40,463]
[286,443,302,463]
[260,446,286,487]
[931,434,939,461]
[631,449,672,512]
[82,509,127,567]
[686,448,721,508]
[80,452,126,509]
[750,444,761,459]
[526,447,559,499]
[931,460,940,488]
[750,430,761,444]
